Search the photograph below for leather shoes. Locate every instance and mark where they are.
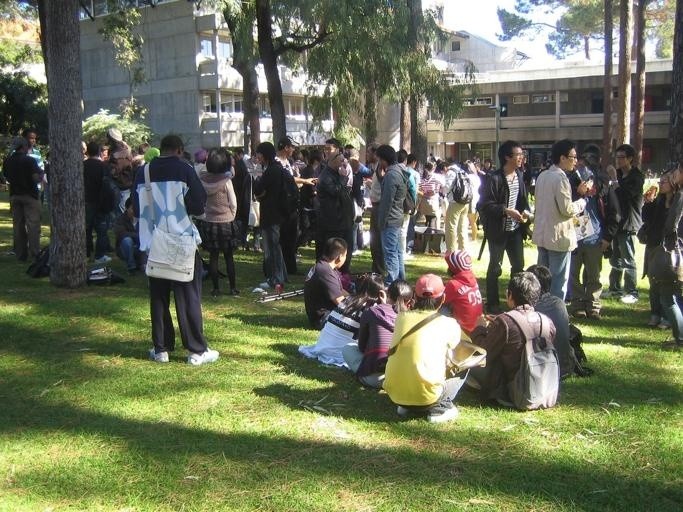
[211,289,218,296]
[230,288,239,294]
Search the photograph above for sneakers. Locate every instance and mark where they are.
[187,348,219,365]
[587,311,600,319]
[599,291,621,298]
[427,405,458,422]
[648,314,671,328]
[94,255,111,263]
[259,282,269,289]
[397,405,408,414]
[573,309,586,317]
[618,293,637,303]
[149,347,169,362]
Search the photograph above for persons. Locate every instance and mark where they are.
[130,135,220,367]
[81,128,160,272]
[341,280,414,389]
[658,188,683,351]
[380,274,471,424]
[477,139,534,312]
[525,265,570,379]
[461,272,557,409]
[534,139,682,330]
[183,136,493,295]
[296,272,386,368]
[2,128,49,263]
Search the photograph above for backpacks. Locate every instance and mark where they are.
[274,164,298,216]
[503,309,560,411]
[445,168,472,204]
[25,247,49,277]
[144,224,196,283]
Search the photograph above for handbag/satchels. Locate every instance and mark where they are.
[247,173,260,228]
[403,191,414,214]
[647,229,682,282]
[447,339,486,378]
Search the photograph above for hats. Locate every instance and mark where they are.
[445,250,471,273]
[278,136,299,146]
[107,128,122,143]
[194,149,205,162]
[416,273,445,298]
